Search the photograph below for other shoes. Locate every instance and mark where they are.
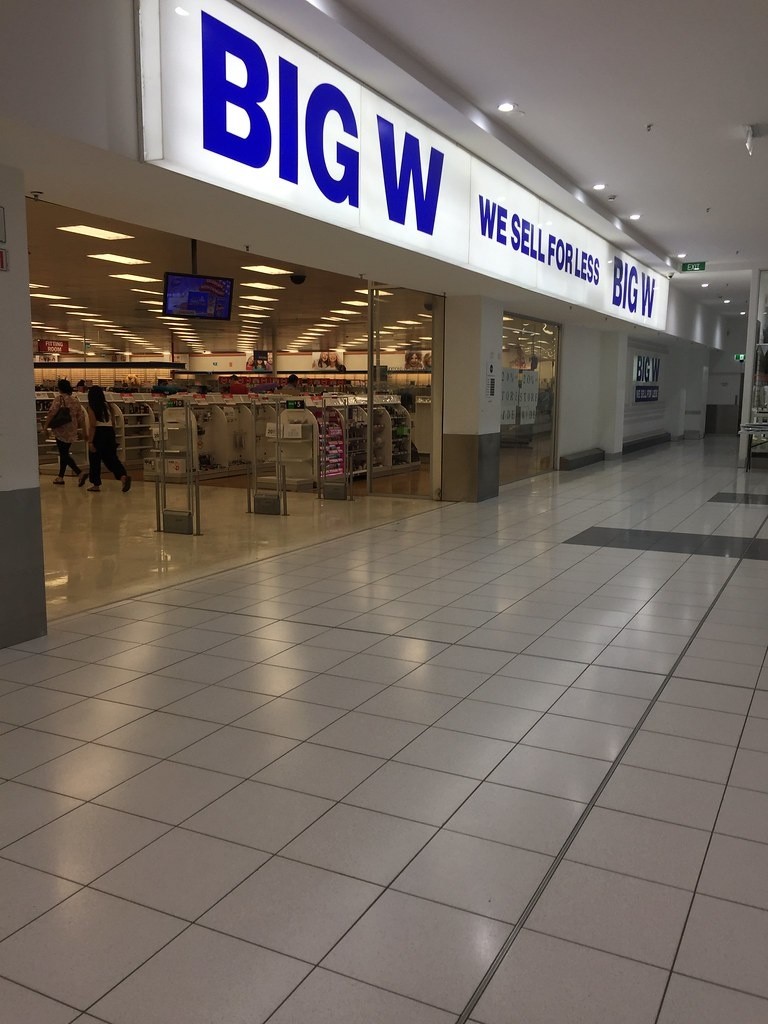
[121,476,131,492]
[87,485,101,492]
[78,471,89,487]
[53,476,65,485]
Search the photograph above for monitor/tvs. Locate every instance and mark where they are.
[254,351,268,360]
[163,272,234,321]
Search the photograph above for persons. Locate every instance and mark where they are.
[312,352,342,369]
[405,350,432,370]
[76,380,93,390]
[224,375,238,390]
[246,353,272,370]
[44,380,88,487]
[281,375,299,391]
[87,385,131,492]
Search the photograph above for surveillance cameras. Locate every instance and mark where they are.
[424,304,433,310]
[668,273,674,278]
[290,276,306,284]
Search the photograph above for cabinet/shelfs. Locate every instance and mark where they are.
[35,381,431,491]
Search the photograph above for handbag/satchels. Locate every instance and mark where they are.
[50,407,72,430]
